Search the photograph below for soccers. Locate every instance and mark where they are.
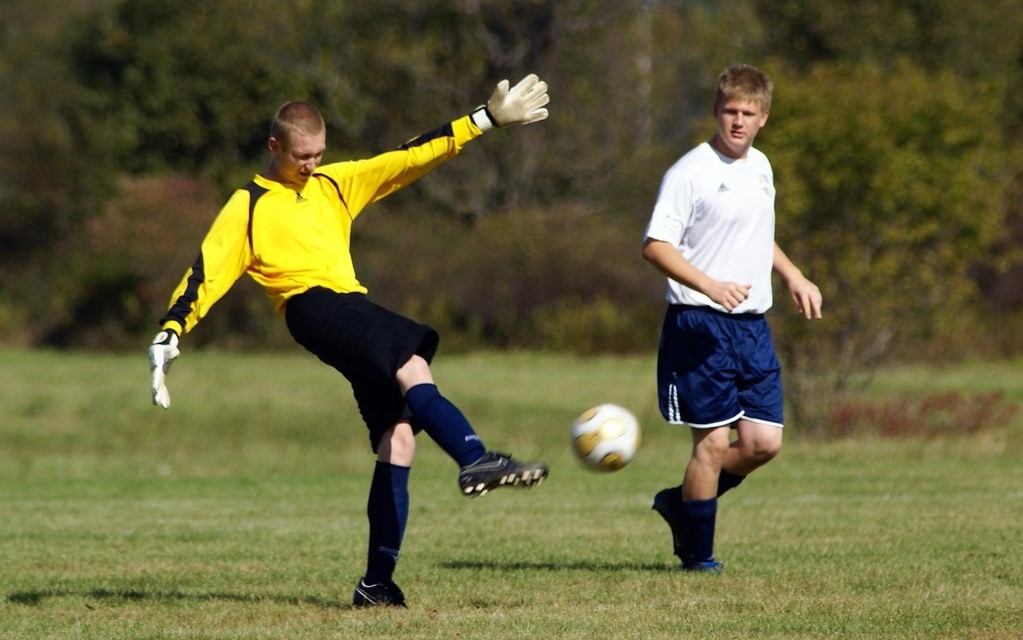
[571,402,643,474]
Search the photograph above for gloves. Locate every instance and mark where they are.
[472,72,550,129]
[148,329,180,409]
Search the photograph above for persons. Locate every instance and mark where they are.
[149,74,551,610]
[638,63,823,571]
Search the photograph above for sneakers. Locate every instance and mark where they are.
[457,451,549,497]
[651,486,686,558]
[352,577,410,610]
[684,562,724,575]
